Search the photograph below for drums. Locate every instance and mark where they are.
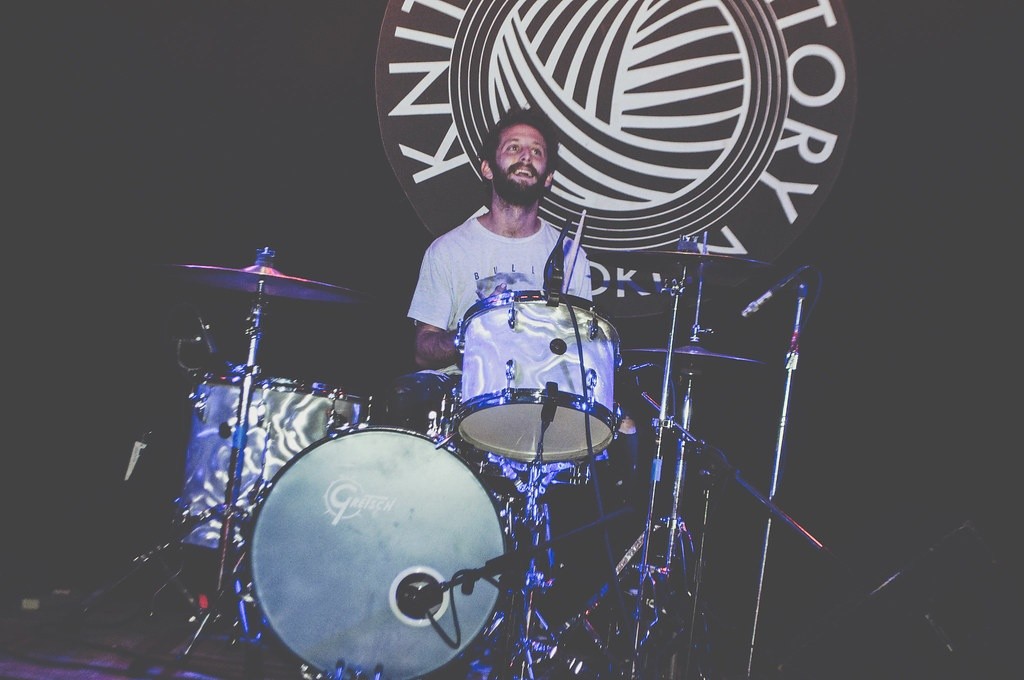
[250,426,506,671]
[455,292,623,464]
[165,371,375,552]
[379,372,487,472]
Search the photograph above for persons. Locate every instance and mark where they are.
[405,108,644,680]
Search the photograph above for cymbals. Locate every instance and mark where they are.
[165,256,375,301]
[641,244,778,273]
[626,346,775,371]
